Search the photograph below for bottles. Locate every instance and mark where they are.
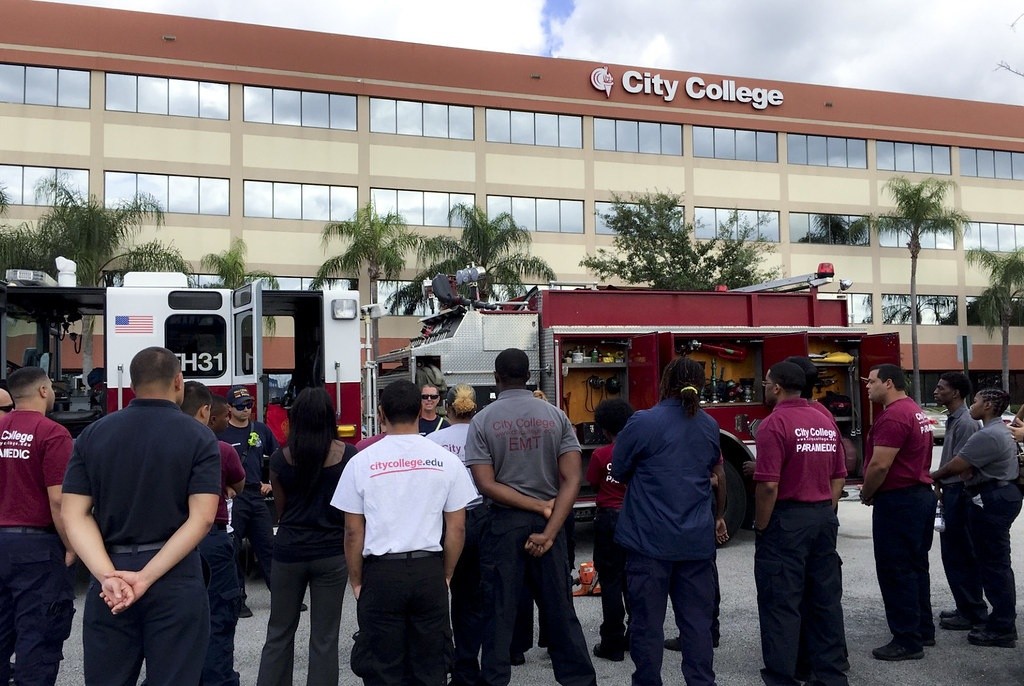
[592,348,598,362]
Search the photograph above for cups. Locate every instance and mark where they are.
[572,353,583,363]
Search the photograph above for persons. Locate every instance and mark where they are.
[664,454,729,651]
[932,372,1024,648]
[419,384,450,436]
[355,403,386,452]
[256,388,360,686]
[753,361,850,686]
[425,384,490,686]
[860,365,935,661]
[587,398,634,660]
[60,348,222,686]
[218,385,307,617]
[611,357,721,686]
[742,356,837,476]
[329,380,477,686]
[0,366,75,686]
[465,349,600,686]
[180,379,247,686]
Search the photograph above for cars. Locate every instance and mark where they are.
[921,407,948,446]
[977,409,1020,439]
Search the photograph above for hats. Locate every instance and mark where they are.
[226,385,254,406]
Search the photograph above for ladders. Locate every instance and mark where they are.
[730,273,818,292]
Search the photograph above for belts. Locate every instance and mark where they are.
[378,551,439,560]
[108,541,164,553]
[0,527,45,534]
[218,524,226,530]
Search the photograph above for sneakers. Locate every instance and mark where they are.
[923,639,936,646]
[872,643,924,660]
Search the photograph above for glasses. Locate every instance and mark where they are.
[421,394,438,400]
[231,402,253,411]
[0,405,15,413]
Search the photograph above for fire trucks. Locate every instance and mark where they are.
[1,256,903,547]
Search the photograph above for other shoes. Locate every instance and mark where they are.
[300,604,307,610]
[238,602,253,618]
[594,644,625,661]
[967,623,1018,647]
[664,638,682,651]
[940,609,989,630]
[510,653,526,666]
